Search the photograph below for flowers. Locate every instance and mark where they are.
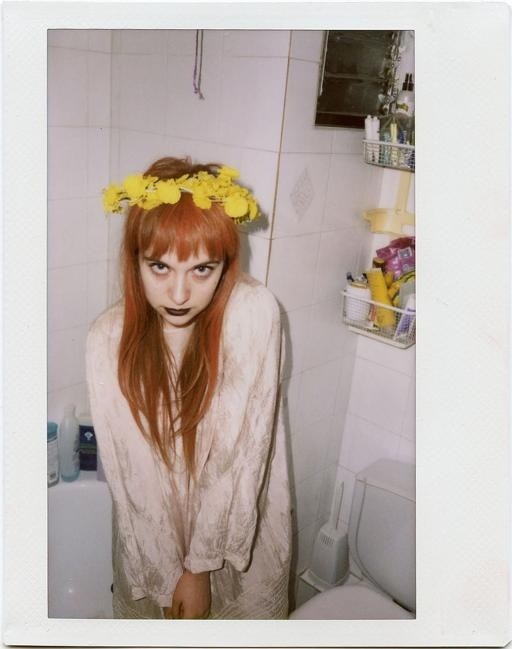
[102,166,263,227]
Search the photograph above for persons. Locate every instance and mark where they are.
[84,155,298,618]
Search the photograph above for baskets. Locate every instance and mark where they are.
[361,138,415,172]
[339,292,415,349]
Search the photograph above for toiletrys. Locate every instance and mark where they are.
[360,69,414,170]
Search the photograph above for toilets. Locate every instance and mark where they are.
[288,458,416,619]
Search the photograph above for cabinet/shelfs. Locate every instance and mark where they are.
[337,135,416,349]
